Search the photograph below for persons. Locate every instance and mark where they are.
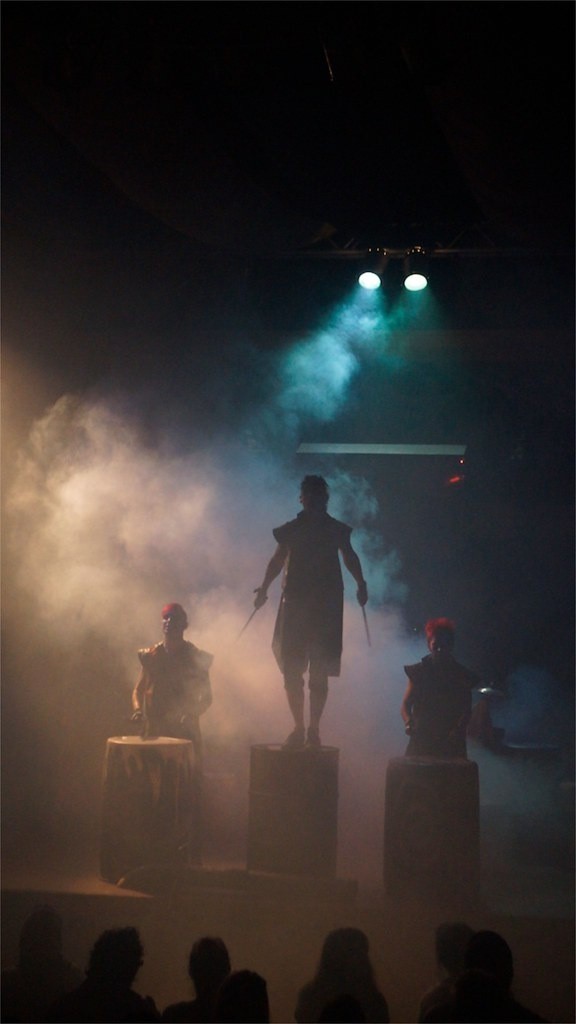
[400,616,478,758]
[132,603,212,866]
[0,903,550,1024]
[253,476,368,750]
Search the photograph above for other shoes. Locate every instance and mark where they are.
[280,726,305,750]
[300,728,322,751]
